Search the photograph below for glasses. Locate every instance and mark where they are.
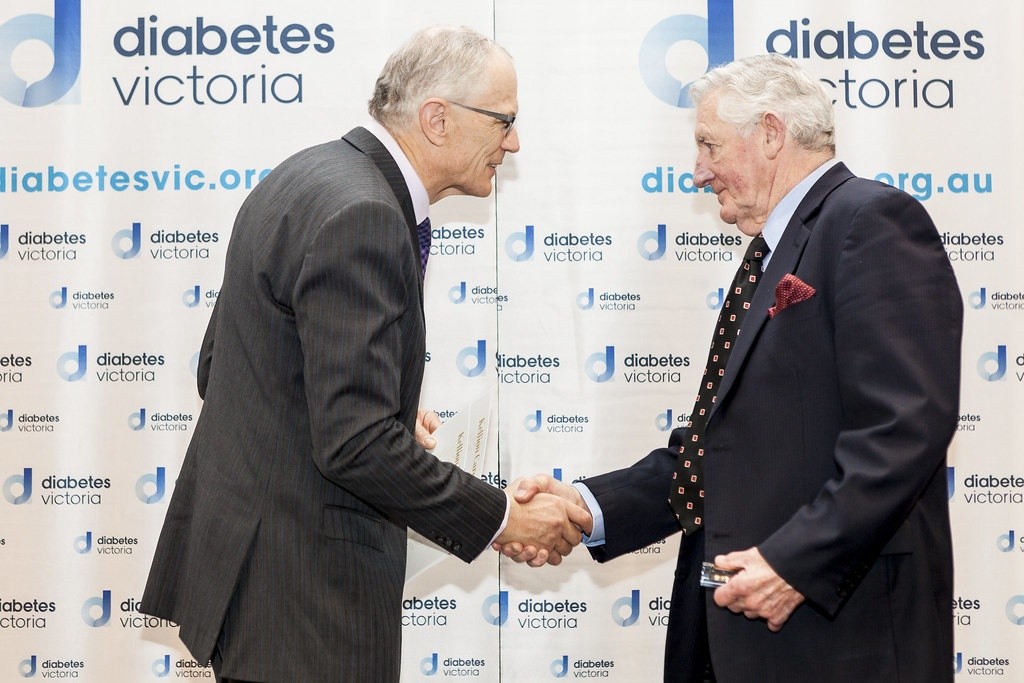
[447,98,517,139]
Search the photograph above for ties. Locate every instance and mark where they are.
[417,216,431,284]
[667,232,772,535]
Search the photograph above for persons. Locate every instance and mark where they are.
[139,24,593,682]
[493,52,963,682]
